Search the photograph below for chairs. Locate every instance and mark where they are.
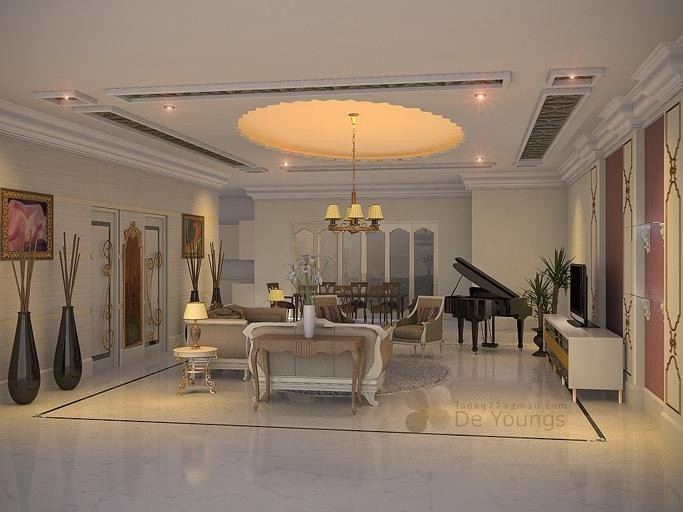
[392,295,446,357]
[183,304,290,381]
[293,281,401,326]
[267,282,297,322]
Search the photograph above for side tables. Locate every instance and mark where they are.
[172,346,218,397]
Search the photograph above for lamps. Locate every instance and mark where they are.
[267,289,286,307]
[324,112,385,234]
[183,301,208,350]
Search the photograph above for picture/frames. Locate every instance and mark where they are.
[0,188,54,260]
[181,213,205,258]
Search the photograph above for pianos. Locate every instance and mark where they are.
[444,258,533,353]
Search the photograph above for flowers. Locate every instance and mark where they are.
[285,254,332,304]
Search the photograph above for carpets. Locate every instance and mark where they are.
[272,348,452,399]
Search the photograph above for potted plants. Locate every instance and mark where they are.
[515,271,559,357]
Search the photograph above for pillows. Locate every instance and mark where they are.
[417,306,439,325]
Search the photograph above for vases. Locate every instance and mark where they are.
[184,290,200,342]
[53,305,82,391]
[211,287,222,305]
[8,312,41,405]
[303,304,315,339]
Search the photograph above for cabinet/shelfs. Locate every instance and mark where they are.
[542,313,624,404]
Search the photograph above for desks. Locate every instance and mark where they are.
[292,292,409,324]
[250,334,366,416]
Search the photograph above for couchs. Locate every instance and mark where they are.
[247,321,394,407]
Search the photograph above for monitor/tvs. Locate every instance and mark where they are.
[567,263,598,328]
